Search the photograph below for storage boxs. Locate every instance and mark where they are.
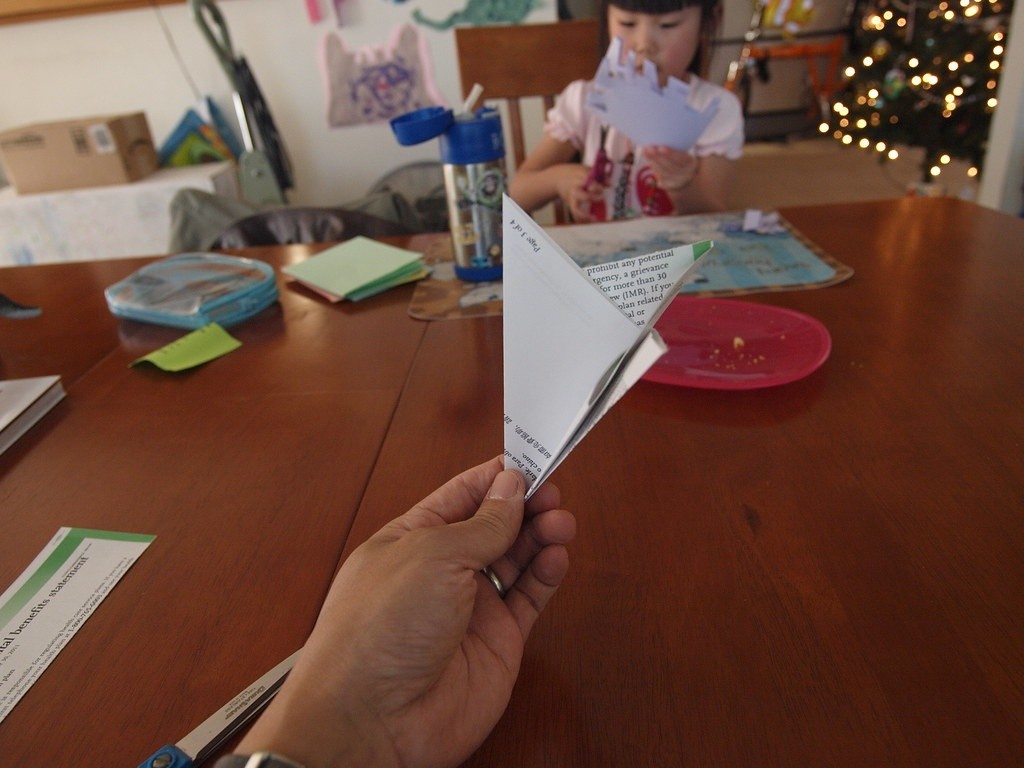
[0,111,159,195]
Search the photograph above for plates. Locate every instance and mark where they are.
[637,296,833,389]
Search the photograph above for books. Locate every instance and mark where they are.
[0,376,66,456]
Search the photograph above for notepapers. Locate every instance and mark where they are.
[285,233,434,304]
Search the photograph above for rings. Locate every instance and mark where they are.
[484,567,506,599]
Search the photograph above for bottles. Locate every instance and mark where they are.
[389,84,511,283]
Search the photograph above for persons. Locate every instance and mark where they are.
[210,450,576,768]
[509,0,744,225]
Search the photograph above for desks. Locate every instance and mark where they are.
[1,161,238,266]
[0,197,1024,768]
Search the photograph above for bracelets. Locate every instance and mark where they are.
[216,751,303,768]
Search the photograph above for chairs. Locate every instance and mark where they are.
[456,17,712,222]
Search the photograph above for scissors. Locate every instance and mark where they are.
[133,647,305,768]
[584,124,614,191]
